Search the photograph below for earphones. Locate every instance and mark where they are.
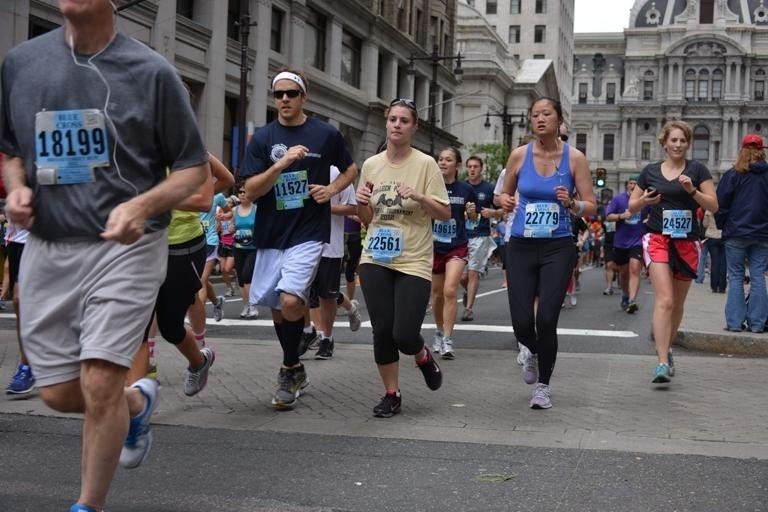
[559,121,562,125]
[108,0,118,12]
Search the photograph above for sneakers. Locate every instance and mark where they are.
[367,328,456,417]
[212,296,260,322]
[516,341,553,409]
[650,344,675,383]
[183,342,215,396]
[118,378,160,469]
[344,300,362,331]
[271,321,335,411]
[4,363,38,394]
[620,295,640,314]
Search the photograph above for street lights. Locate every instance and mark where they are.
[405,41,465,155]
[481,105,527,170]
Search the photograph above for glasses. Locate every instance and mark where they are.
[387,98,416,114]
[275,90,301,98]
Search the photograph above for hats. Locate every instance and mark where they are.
[743,135,768,149]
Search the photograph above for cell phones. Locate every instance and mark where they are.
[647,186,658,198]
[359,181,375,207]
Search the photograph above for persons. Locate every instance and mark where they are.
[355,98,451,418]
[1,0,209,512]
[498,96,596,409]
[0,133,767,397]
[627,118,719,390]
[235,67,358,410]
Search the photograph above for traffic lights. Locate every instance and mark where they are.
[596,168,605,187]
